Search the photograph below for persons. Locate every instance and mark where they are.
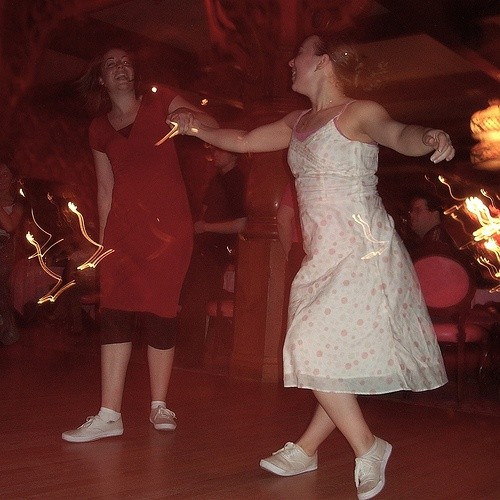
[0,114,257,372]
[165,31,456,500]
[58,42,219,443]
[393,194,475,336]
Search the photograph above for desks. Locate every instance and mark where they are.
[9,264,65,332]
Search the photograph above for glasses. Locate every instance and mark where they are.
[409,208,429,215]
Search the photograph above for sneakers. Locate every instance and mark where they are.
[354,434,393,500]
[61,415,124,443]
[260,441,318,476]
[148,405,178,432]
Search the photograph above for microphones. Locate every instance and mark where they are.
[128,79,135,83]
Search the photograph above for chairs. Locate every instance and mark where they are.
[401,255,491,403]
[202,265,236,369]
[64,259,100,337]
